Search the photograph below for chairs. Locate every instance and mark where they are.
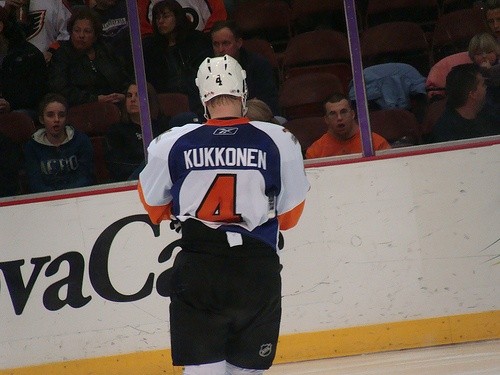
[0,110,36,147]
[224,0,491,161]
[158,93,192,120]
[69,102,123,186]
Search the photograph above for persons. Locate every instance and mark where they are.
[0,1,277,125]
[10,95,95,194]
[468,0,500,79]
[101,80,173,188]
[304,92,391,161]
[137,55,311,374]
[423,63,499,146]
[193,20,275,121]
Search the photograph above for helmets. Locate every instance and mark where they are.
[195,54,248,120]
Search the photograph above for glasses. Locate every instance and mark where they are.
[323,109,354,118]
[156,14,174,20]
